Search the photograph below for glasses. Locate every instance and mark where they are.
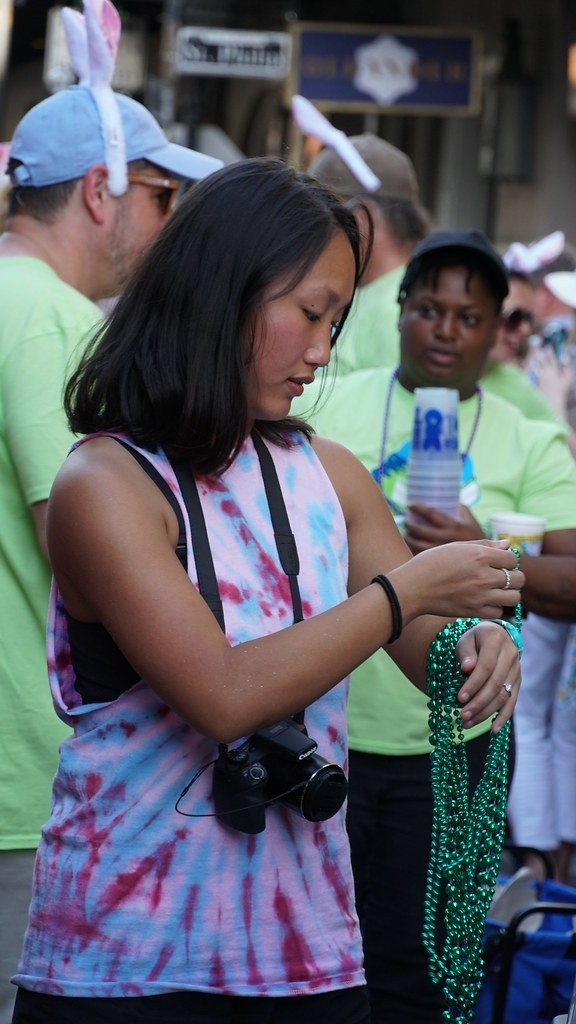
[502,308,534,333]
[125,175,180,214]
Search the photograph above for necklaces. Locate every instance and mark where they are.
[423,544,522,1024]
[378,364,483,514]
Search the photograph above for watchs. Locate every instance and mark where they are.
[491,618,523,661]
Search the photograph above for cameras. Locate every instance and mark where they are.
[212,717,350,835]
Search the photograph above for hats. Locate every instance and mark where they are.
[309,132,418,200]
[406,230,509,296]
[544,267,576,308]
[7,83,223,189]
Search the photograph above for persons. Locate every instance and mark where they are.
[493,240,576,878]
[10,160,524,1023]
[291,231,576,1024]
[0,91,228,1024]
[310,136,576,454]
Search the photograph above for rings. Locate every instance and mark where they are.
[502,567,510,589]
[503,683,512,697]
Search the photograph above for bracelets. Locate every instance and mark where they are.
[370,571,403,645]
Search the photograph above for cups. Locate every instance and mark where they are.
[487,511,546,557]
[407,385,462,521]
